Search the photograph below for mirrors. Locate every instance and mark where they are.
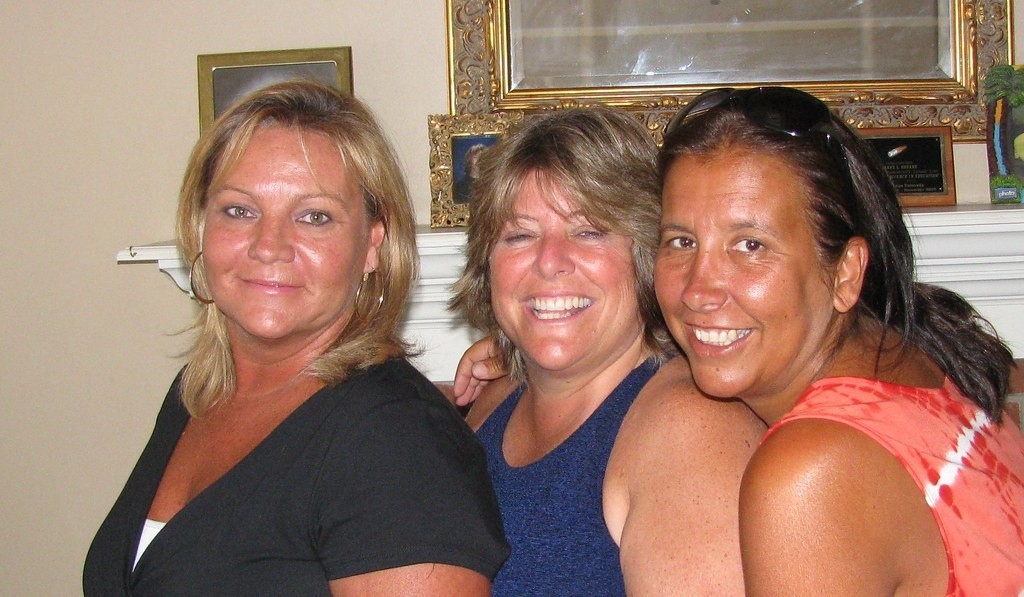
[444,0,1015,146]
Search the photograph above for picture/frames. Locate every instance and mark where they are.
[427,113,529,229]
[197,46,354,136]
[855,125,957,206]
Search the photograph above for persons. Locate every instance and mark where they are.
[82,81,510,597]
[450,87,1024,597]
[453,144,488,204]
[443,105,770,597]
